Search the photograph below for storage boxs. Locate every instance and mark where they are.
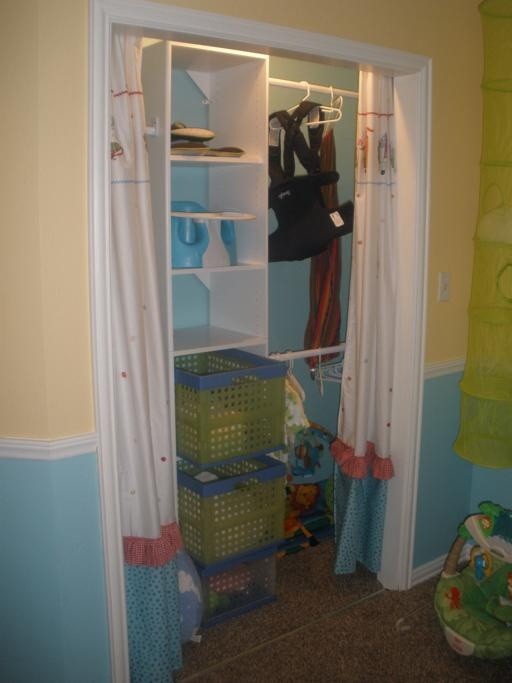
[176,454,288,569]
[189,547,279,628]
[174,347,287,469]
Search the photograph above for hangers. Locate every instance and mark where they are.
[324,85,344,136]
[268,81,343,131]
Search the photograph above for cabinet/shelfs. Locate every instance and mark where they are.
[142,38,394,630]
